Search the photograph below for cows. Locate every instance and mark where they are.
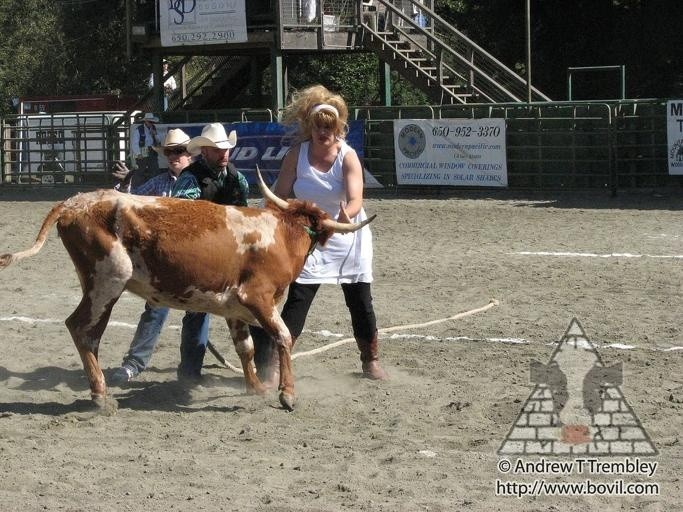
[0,163,378,411]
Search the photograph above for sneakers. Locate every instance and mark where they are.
[112,367,133,382]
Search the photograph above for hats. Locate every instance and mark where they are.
[186,122,238,157]
[150,128,192,158]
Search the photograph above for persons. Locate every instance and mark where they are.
[168,122,273,390]
[128,113,160,172]
[109,128,191,384]
[264,85,384,383]
[146,59,177,112]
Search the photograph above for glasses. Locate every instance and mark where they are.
[163,147,186,156]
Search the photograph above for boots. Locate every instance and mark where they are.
[354,330,383,380]
[261,334,296,390]
